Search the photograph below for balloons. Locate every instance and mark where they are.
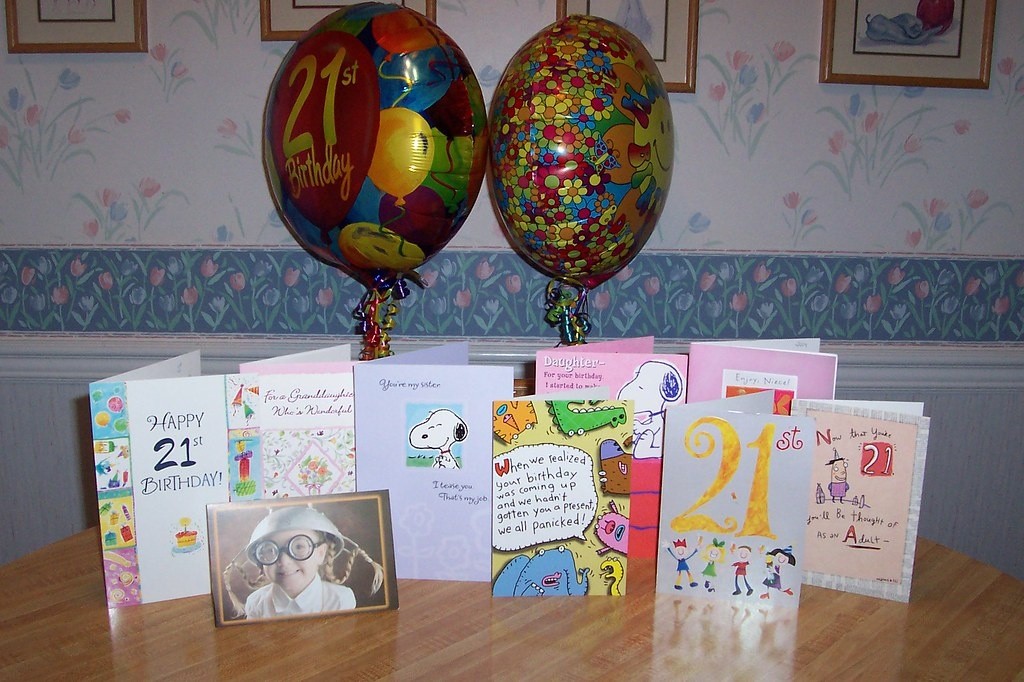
[487,15,675,344]
[262,2,487,362]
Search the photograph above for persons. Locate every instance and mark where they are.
[223,506,383,619]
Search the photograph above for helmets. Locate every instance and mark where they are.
[245,507,345,569]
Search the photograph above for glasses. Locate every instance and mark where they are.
[252,534,326,566]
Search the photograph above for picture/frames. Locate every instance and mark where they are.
[5,0,148,53]
[556,0,699,94]
[818,0,997,90]
[259,0,436,42]
[207,489,399,627]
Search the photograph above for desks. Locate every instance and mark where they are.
[0,528,1024,682]
